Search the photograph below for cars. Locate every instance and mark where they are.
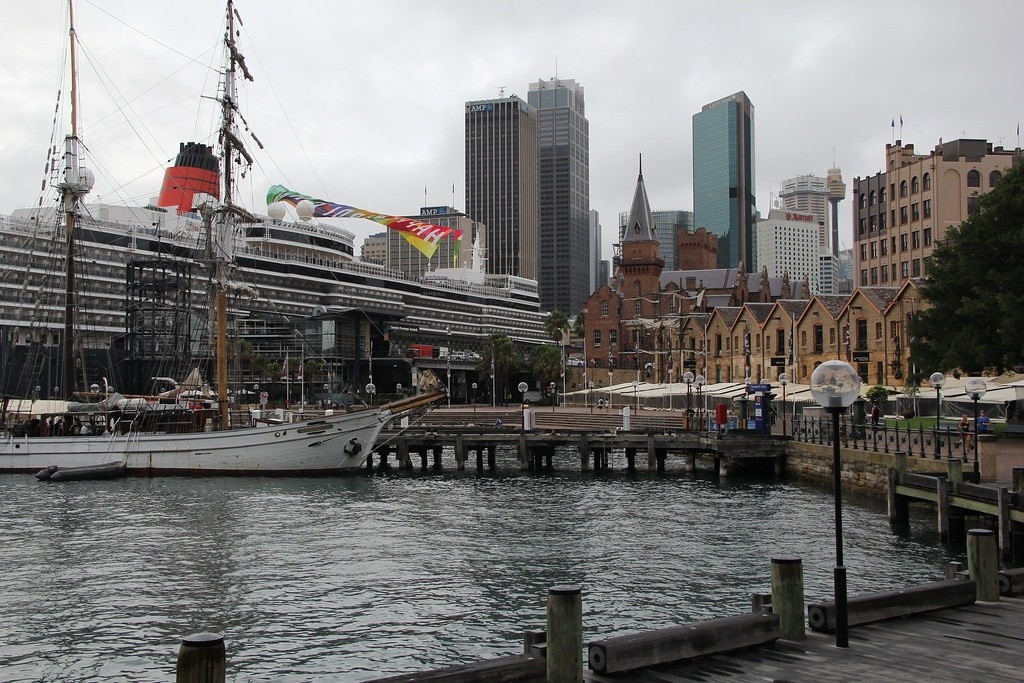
[560,354,588,367]
[406,344,485,362]
[644,361,655,370]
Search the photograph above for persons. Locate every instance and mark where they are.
[959,413,971,443]
[690,406,695,417]
[598,397,603,408]
[80,423,87,435]
[49,418,62,436]
[496,416,502,425]
[871,402,880,426]
[605,398,609,409]
[322,397,337,410]
[977,410,991,430]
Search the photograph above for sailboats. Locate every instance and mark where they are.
[1,1,451,476]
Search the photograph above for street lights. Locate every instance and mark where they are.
[695,375,705,431]
[396,383,402,400]
[518,381,528,434]
[472,383,478,411]
[588,382,594,413]
[550,381,556,412]
[778,373,790,436]
[683,372,694,433]
[810,359,862,649]
[254,384,260,409]
[365,383,376,407]
[965,378,987,484]
[929,372,946,459]
[744,377,752,419]
